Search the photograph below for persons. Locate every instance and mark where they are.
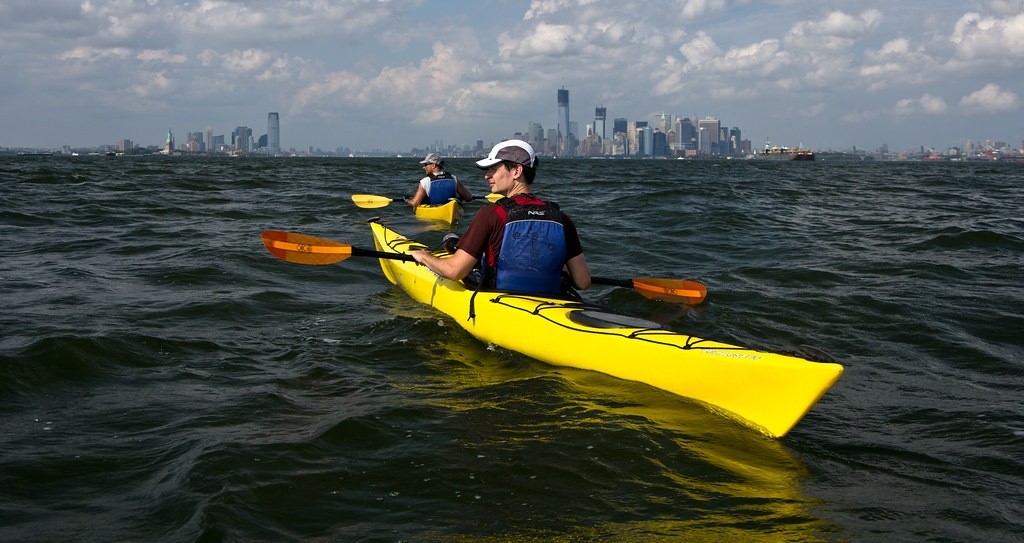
[412,138,593,298]
[402,152,473,207]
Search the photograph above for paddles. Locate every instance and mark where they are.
[261,228,707,305]
[352,192,508,210]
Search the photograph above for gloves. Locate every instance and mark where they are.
[402,195,409,202]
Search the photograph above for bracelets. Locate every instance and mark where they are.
[406,200,411,204]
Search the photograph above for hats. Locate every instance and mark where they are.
[474,139,536,170]
[420,153,445,167]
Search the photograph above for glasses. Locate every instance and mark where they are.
[424,163,432,167]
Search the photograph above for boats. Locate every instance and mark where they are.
[752,137,817,161]
[413,193,462,226]
[367,215,847,440]
